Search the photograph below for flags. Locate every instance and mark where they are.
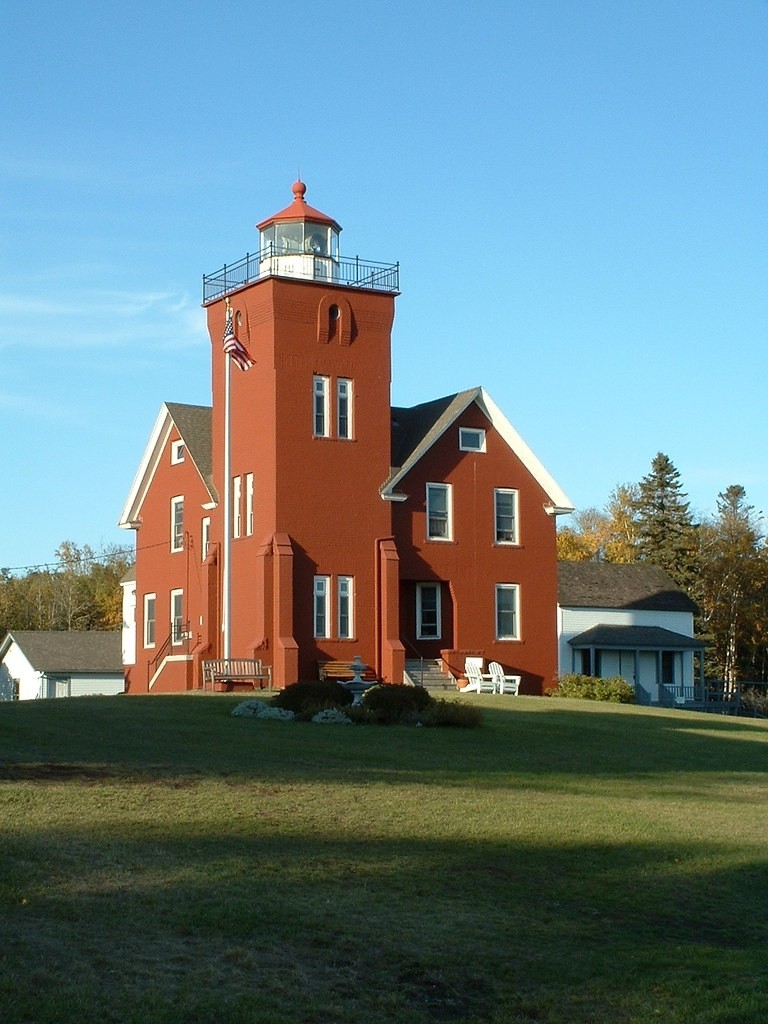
[223,320,256,372]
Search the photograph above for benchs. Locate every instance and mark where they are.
[315,660,367,682]
[202,659,272,693]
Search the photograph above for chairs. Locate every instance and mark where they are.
[462,662,497,695]
[487,662,522,697]
